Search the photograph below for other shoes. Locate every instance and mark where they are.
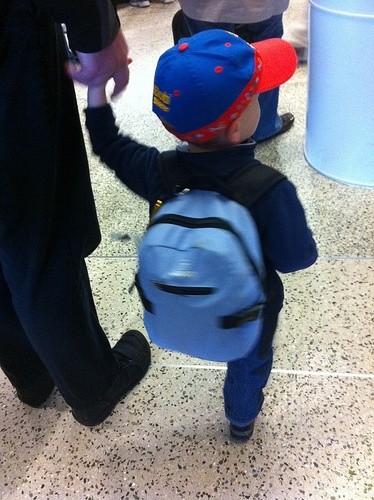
[15,371,57,409]
[229,420,254,442]
[70,329,152,427]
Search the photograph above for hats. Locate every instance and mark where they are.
[152,28,299,144]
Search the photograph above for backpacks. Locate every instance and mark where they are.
[136,149,289,363]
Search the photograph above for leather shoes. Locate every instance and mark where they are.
[255,113,295,145]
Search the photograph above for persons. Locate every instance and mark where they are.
[84,29,318,443]
[171,1,298,146]
[0,0,152,426]
